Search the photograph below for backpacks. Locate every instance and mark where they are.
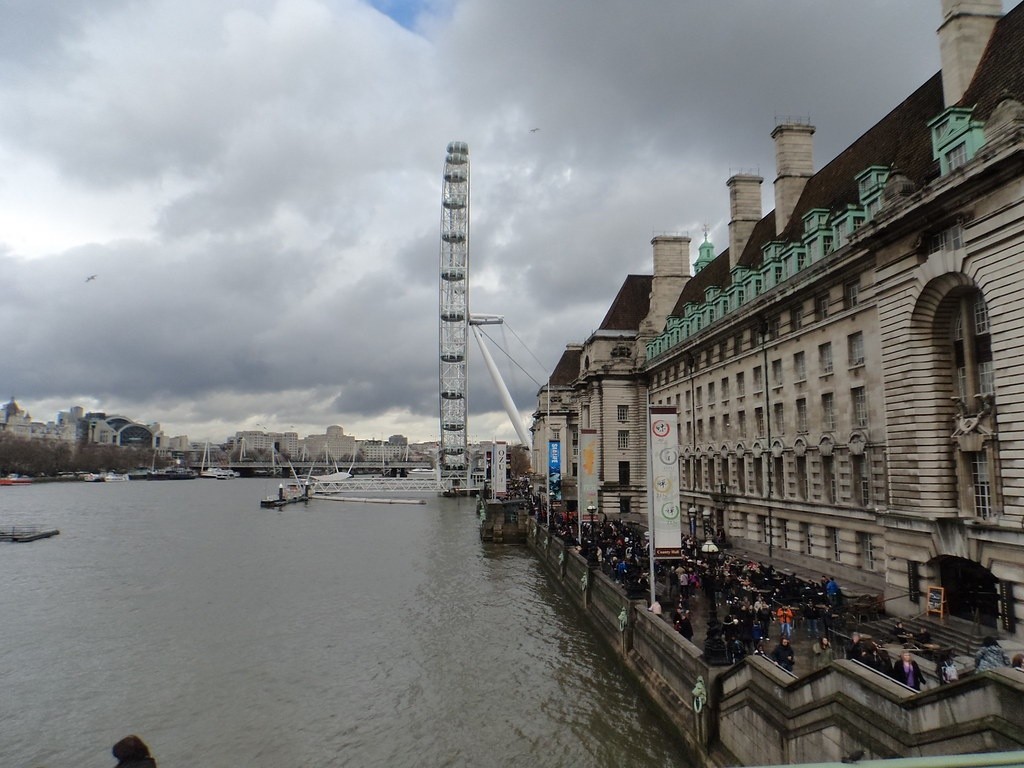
[944,661,958,680]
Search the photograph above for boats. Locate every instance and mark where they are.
[83,472,130,483]
[0,473,33,486]
[144,439,241,481]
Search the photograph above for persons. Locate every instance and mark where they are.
[508,480,1024,696]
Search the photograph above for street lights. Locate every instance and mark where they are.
[689,507,697,539]
[528,484,536,517]
[537,490,544,524]
[548,491,556,534]
[585,505,600,567]
[702,539,734,666]
[703,506,714,541]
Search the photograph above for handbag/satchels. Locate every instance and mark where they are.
[696,581,699,588]
